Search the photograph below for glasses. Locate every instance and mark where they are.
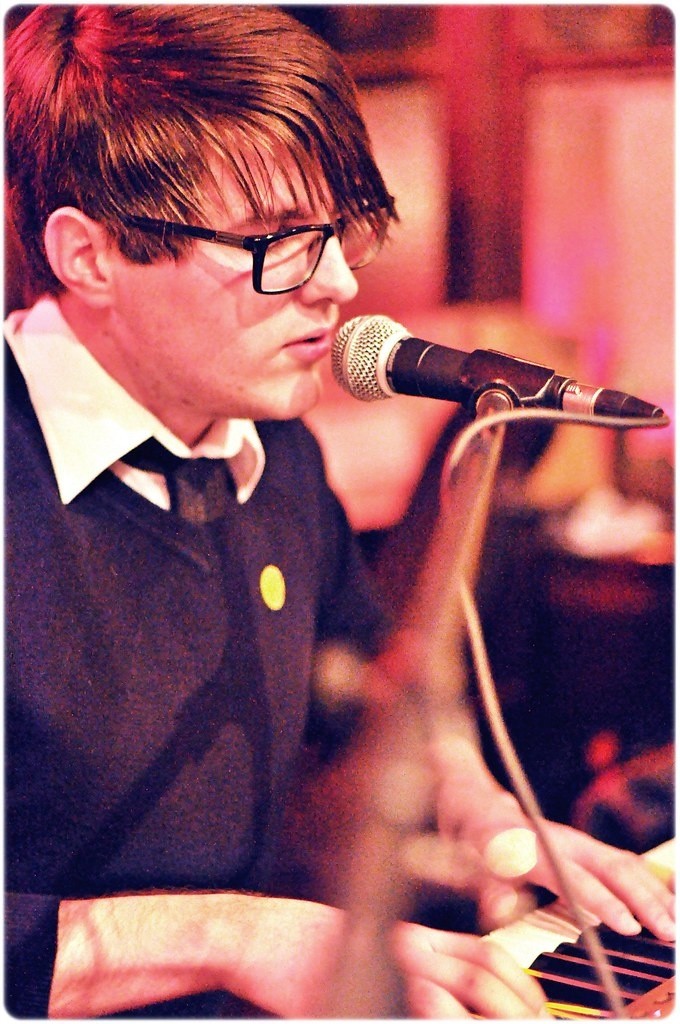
[119,189,392,296]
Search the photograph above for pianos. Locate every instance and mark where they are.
[466,835,676,1019]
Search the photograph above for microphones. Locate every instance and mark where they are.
[329,315,667,429]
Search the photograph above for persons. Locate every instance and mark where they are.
[4,4,674,1020]
